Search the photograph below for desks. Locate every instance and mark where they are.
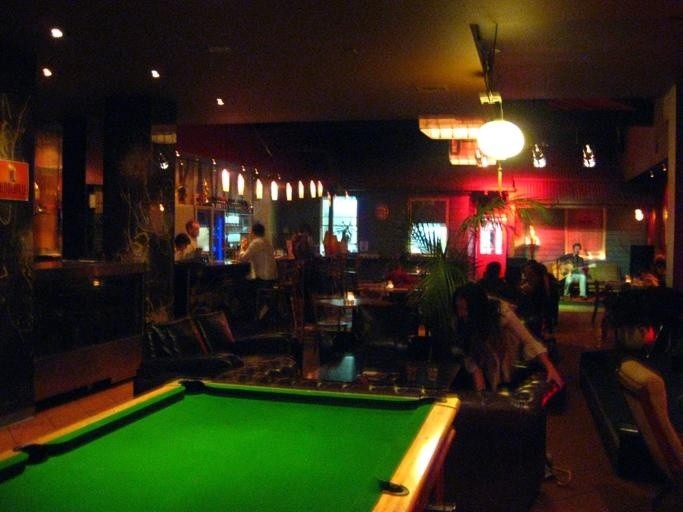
[0,375,464,512]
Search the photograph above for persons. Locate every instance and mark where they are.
[643,254,665,288]
[173,233,190,261]
[557,243,587,301]
[290,233,314,267]
[185,221,200,259]
[446,258,564,392]
[239,222,279,321]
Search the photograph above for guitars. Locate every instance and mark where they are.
[553,262,597,280]
